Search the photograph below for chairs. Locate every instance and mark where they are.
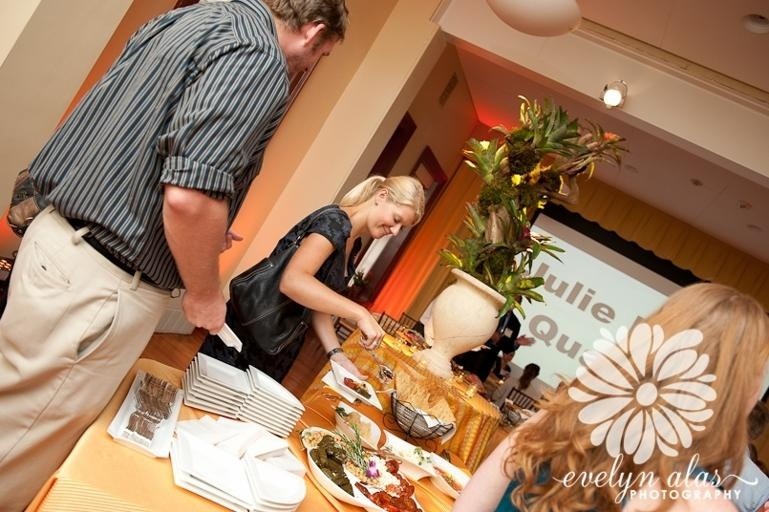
[500,387,538,416]
[398,313,417,332]
[378,312,401,338]
[315,318,354,355]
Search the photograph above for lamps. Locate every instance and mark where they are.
[599,79,629,110]
[480,0,581,37]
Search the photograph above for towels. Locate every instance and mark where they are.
[168,415,306,512]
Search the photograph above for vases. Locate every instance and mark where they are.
[411,264,513,380]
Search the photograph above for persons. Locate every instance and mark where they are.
[0,0,349,512]
[186,175,425,384]
[451,283,769,512]
[452,295,540,409]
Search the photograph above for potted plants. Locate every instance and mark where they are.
[346,272,370,301]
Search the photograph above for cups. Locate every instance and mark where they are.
[465,383,480,397]
[408,339,422,354]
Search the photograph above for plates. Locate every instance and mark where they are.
[329,359,384,413]
[107,351,306,512]
[303,401,470,512]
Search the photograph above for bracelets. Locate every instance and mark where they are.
[326,348,344,359]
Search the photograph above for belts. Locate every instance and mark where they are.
[69,219,156,285]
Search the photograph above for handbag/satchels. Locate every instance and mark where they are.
[230,208,349,356]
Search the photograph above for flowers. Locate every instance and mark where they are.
[431,94,629,338]
